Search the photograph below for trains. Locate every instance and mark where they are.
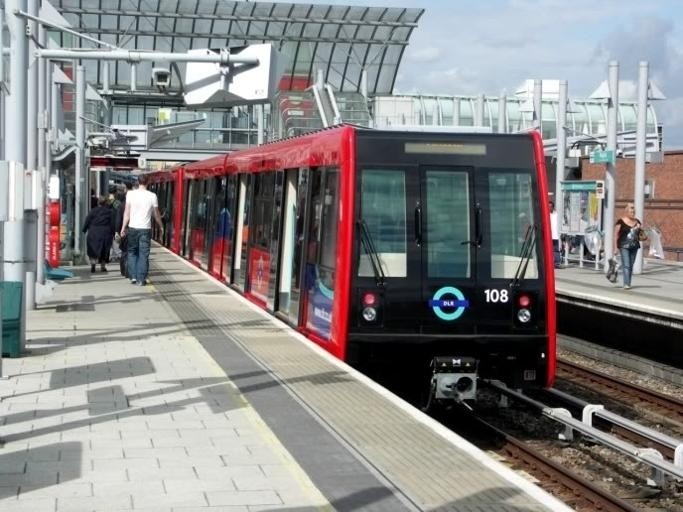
[137,124,556,422]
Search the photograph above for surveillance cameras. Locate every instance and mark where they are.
[151,66,171,87]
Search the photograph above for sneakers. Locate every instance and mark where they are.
[91,264,106,273]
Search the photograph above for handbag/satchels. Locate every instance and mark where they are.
[607,258,620,282]
[639,230,647,241]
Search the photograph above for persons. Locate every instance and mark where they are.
[84,186,128,278]
[548,202,562,269]
[613,202,647,290]
[121,173,164,287]
